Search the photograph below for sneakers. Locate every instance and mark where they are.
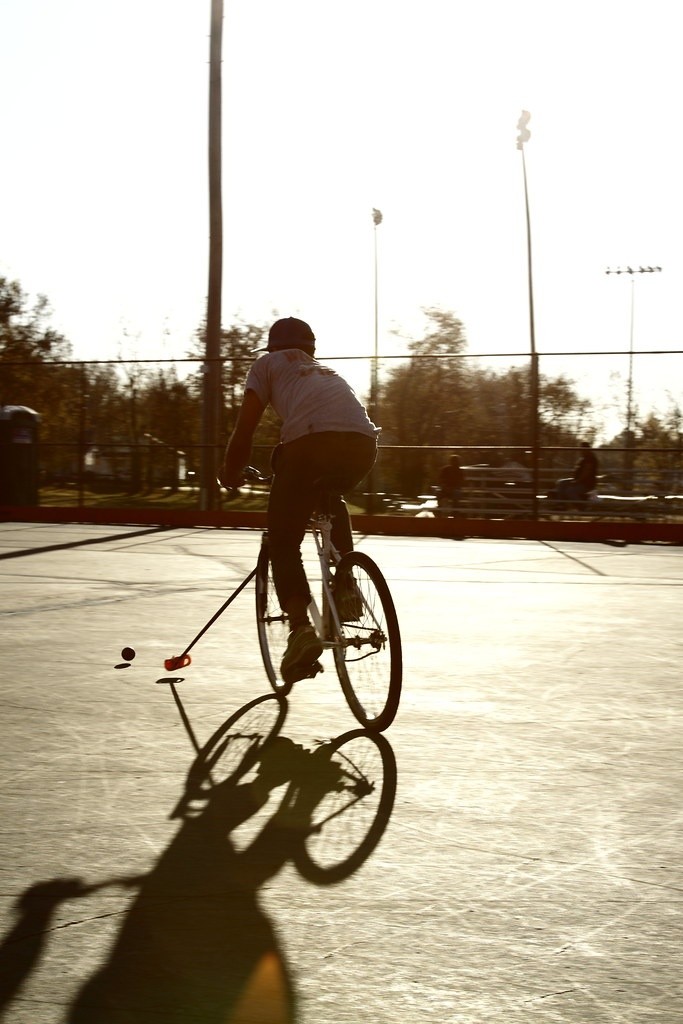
[333,578,363,619]
[280,624,322,683]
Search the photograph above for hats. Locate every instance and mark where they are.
[249,317,317,353]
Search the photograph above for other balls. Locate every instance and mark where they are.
[121,646,135,661]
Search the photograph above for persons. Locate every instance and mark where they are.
[437,455,464,515]
[219,317,382,684]
[565,442,598,511]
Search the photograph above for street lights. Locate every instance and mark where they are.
[515,111,540,519]
[605,266,661,427]
[372,205,382,398]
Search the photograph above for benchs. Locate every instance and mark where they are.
[423,467,683,522]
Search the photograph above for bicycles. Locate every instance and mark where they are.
[242,466,403,732]
